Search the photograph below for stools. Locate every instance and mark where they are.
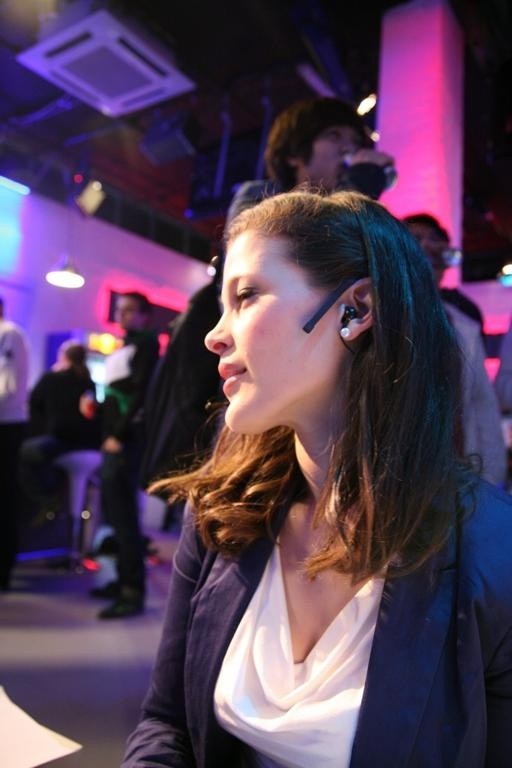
[41,447,107,575]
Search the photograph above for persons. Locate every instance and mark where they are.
[120,190,512,767]
[0,279,226,617]
[400,211,511,495]
[227,96,394,233]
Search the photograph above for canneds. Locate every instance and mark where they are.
[441,249,463,267]
[343,153,397,188]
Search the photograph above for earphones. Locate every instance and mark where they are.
[341,305,357,327]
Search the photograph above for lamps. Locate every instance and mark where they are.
[46,158,87,289]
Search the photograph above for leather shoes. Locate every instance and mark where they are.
[97,597,145,619]
[89,580,119,599]
